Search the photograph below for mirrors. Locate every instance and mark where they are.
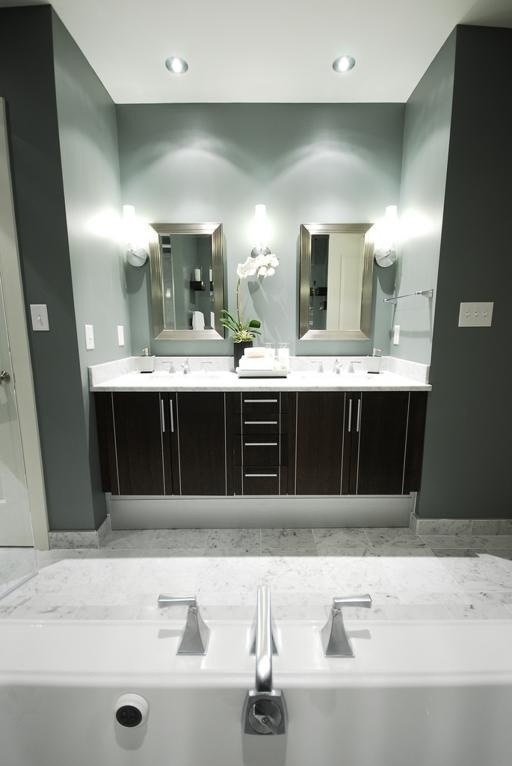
[296,220,378,346]
[141,218,230,346]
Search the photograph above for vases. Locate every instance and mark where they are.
[234,343,254,373]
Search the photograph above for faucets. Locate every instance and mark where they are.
[180,357,194,375]
[245,585,290,738]
[333,360,342,375]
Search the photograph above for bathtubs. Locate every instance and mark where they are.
[3,612,510,761]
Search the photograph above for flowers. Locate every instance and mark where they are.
[221,255,278,342]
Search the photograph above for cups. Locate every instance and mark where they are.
[264,341,291,370]
[368,354,382,373]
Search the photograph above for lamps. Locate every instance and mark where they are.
[375,202,401,268]
[115,203,148,269]
[249,203,272,258]
